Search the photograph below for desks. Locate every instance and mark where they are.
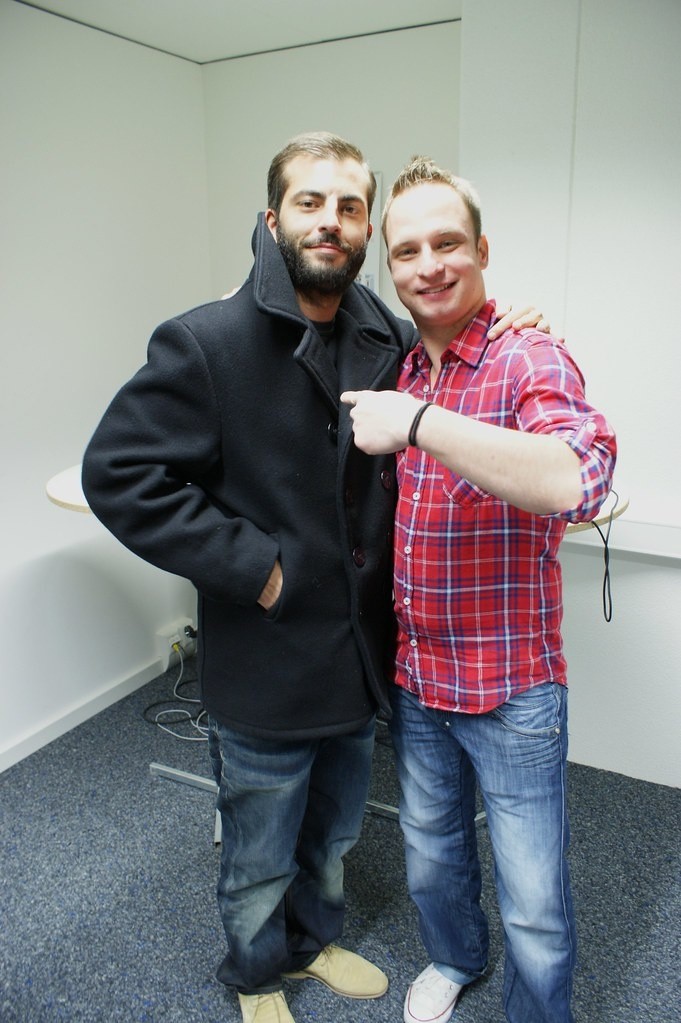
[45,464,629,843]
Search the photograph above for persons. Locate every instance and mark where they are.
[83,131,566,1022]
[340,157,616,1022]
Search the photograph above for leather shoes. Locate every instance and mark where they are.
[237,986,296,1023]
[278,943,389,999]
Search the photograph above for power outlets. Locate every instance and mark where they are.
[156,616,196,672]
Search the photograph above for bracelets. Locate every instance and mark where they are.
[408,403,433,447]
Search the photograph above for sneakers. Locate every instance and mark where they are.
[403,961,464,1023]
